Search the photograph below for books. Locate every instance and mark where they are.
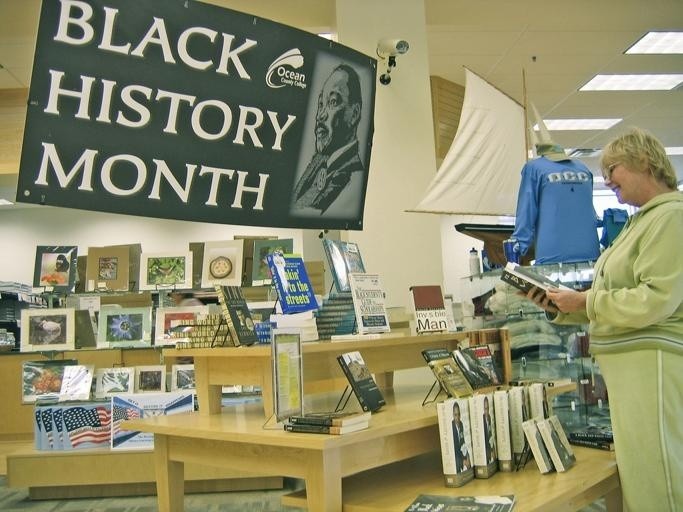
[283,410,372,435]
[405,286,575,512]
[172,239,391,349]
[337,351,385,413]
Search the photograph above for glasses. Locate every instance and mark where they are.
[602,162,623,179]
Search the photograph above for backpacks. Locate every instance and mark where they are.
[598,208,629,248]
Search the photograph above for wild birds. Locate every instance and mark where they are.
[39,319,63,337]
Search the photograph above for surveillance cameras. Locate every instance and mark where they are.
[376,39,409,80]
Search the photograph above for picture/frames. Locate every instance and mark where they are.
[58,357,195,406]
[32,238,292,292]
[20,306,208,350]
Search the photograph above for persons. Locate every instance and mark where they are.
[507,141,601,268]
[516,130,683,511]
[291,65,366,219]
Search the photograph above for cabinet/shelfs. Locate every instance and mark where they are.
[1,330,286,502]
[121,337,618,512]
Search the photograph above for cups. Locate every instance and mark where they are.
[503,239,519,263]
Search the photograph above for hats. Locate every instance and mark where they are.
[536,142,572,163]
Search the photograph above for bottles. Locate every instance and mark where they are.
[469,247,480,274]
